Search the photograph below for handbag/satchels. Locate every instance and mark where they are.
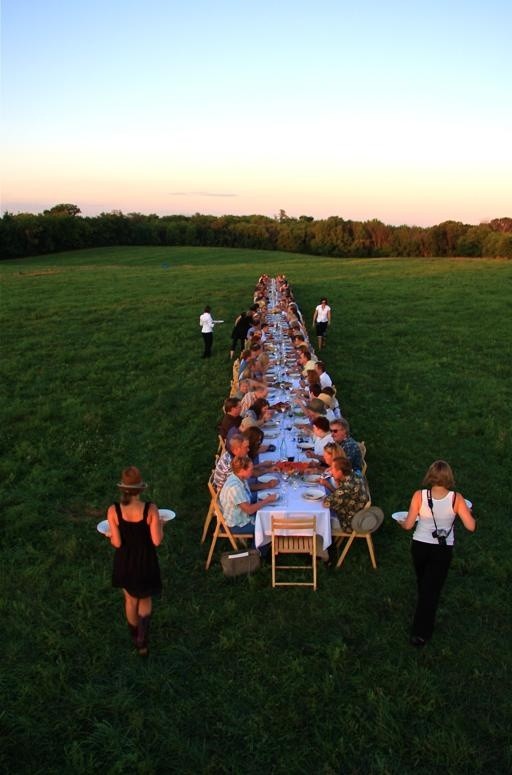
[220,549,260,576]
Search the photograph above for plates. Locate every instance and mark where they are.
[213,320,224,323]
[463,498,472,509]
[293,406,325,505]
[390,511,420,522]
[255,474,282,502]
[157,508,177,522]
[96,519,111,535]
[262,321,303,440]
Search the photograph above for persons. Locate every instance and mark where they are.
[213,273,366,567]
[396,459,478,649]
[199,305,215,358]
[105,465,166,655]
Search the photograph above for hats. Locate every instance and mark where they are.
[116,467,148,489]
[304,392,331,414]
[351,507,384,533]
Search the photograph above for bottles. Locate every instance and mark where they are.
[276,371,300,492]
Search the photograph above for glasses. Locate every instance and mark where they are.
[329,428,343,433]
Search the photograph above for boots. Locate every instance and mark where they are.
[127,614,151,654]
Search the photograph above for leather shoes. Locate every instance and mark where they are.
[323,544,338,568]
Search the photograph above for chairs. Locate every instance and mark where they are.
[270,513,317,592]
[330,441,375,571]
[221,278,337,560]
[202,433,254,571]
